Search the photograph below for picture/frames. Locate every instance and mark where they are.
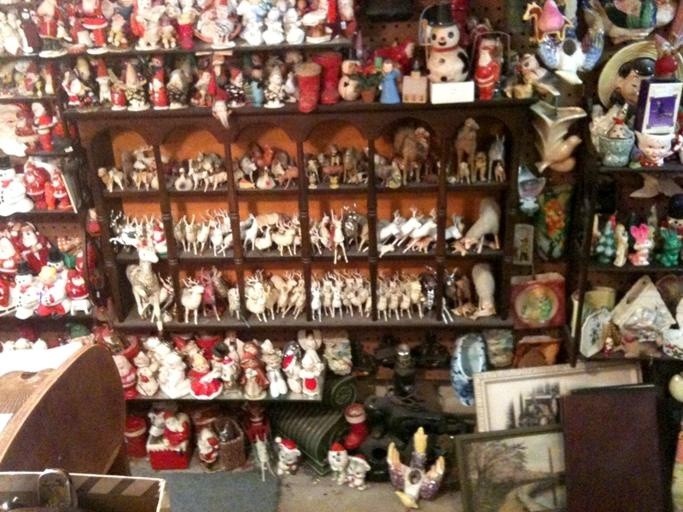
[452,424,568,512]
[472,358,644,431]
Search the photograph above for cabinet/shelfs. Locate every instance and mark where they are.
[560,155,681,369]
[1,0,519,419]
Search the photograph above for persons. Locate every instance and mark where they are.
[0,0,355,210]
[609,56,657,118]
[0,2,680,511]
